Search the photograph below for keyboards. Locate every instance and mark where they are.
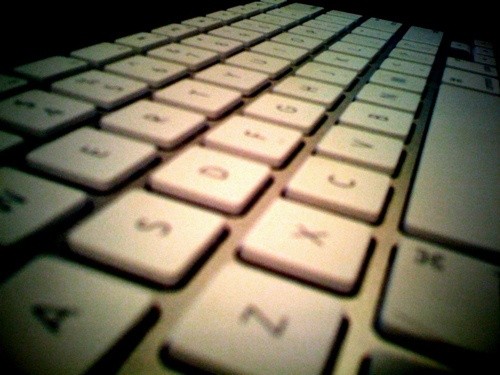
[0,3,499,375]
